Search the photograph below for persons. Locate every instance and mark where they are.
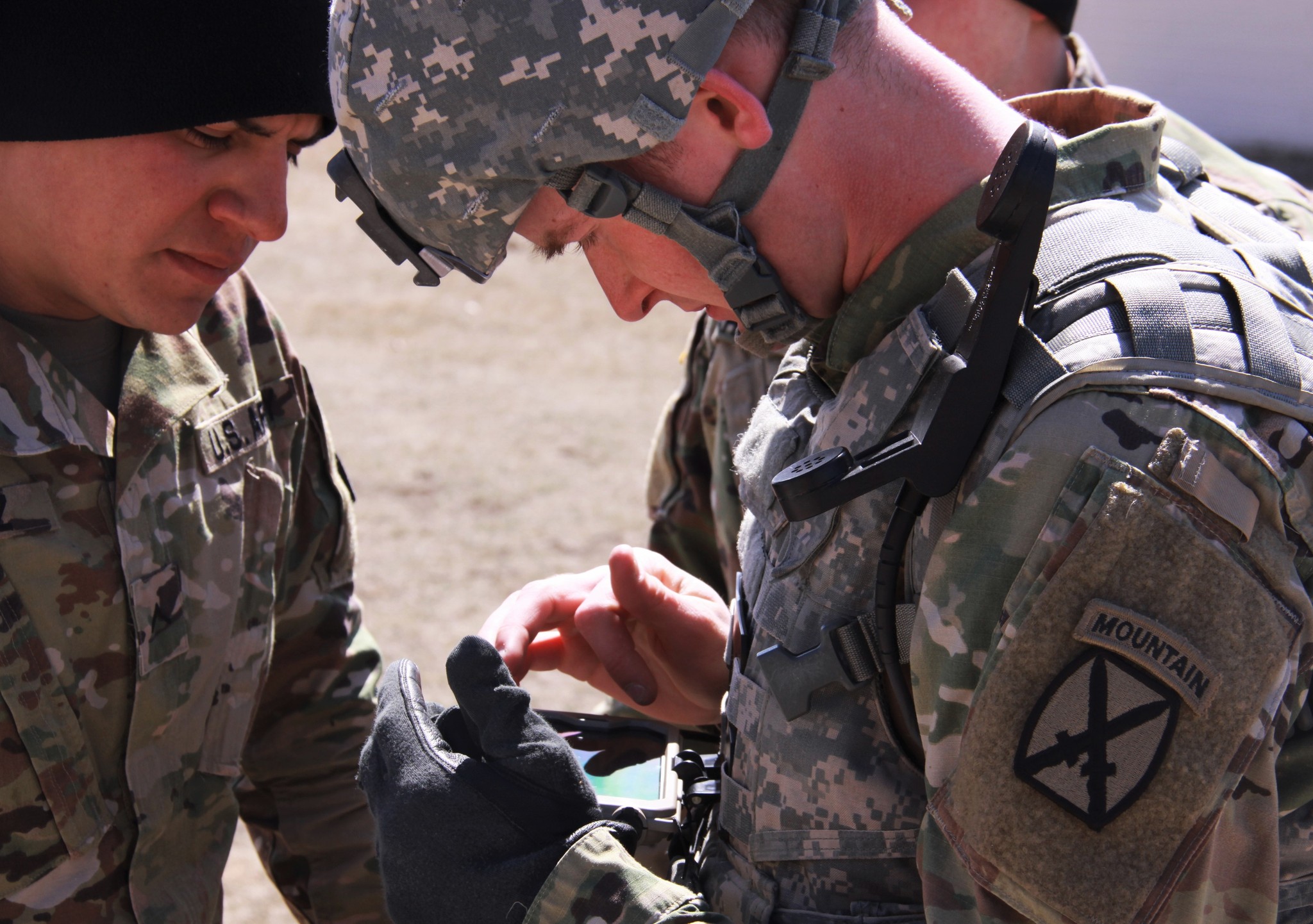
[0,0,389,924]
[328,0,1313,923]
[598,0,1313,751]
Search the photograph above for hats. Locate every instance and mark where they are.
[0,0,338,148]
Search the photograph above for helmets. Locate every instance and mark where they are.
[326,0,766,284]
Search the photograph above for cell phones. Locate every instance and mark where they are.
[542,719,678,818]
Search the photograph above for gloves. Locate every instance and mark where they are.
[355,635,645,924]
